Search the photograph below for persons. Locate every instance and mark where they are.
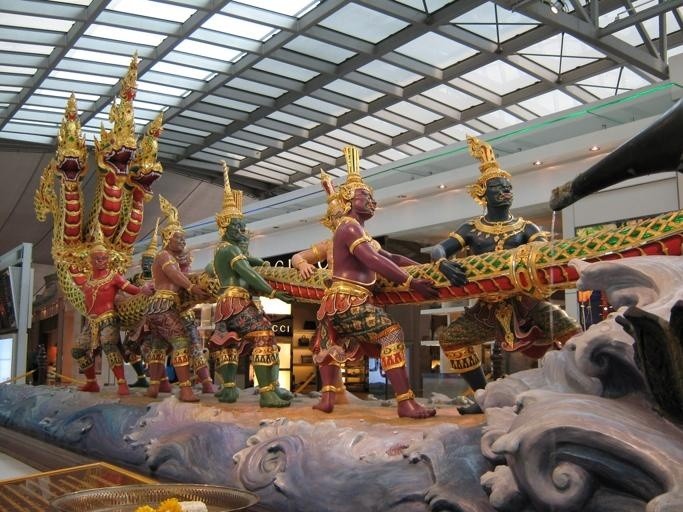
[214,159,293,408]
[290,237,428,398]
[152,248,214,394]
[202,232,295,401]
[128,217,167,389]
[138,193,208,403]
[430,131,584,416]
[312,147,439,417]
[70,243,140,394]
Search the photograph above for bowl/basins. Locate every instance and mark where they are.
[52,483,260,512]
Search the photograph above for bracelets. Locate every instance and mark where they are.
[404,276,412,288]
[434,257,448,270]
[187,283,195,294]
[269,289,277,299]
[295,259,308,266]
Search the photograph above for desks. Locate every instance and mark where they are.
[0,428,275,512]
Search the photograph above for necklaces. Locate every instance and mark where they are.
[86,269,115,288]
[467,216,526,243]
[334,217,361,231]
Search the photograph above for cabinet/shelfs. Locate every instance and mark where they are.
[419,304,494,396]
[292,302,368,393]
